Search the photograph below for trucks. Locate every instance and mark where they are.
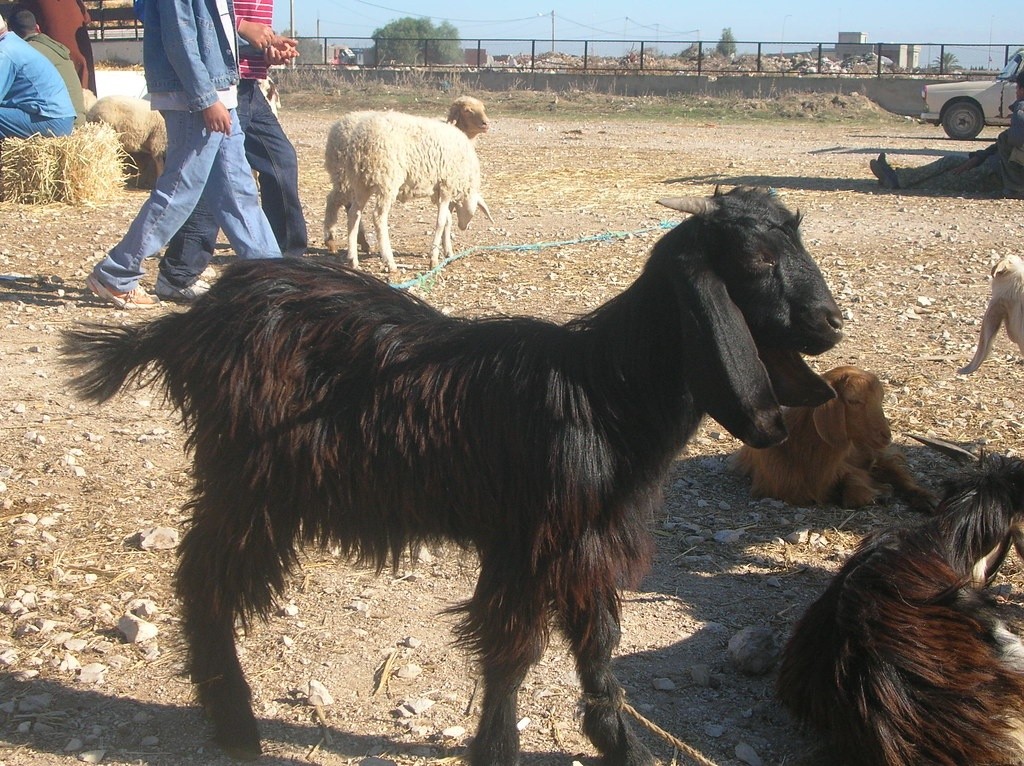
[329,43,357,66]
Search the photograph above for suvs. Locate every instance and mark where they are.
[919,46,1024,141]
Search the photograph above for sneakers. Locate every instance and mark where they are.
[156,270,211,301]
[85,272,161,310]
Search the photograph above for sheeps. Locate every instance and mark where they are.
[321,96,490,255]
[82,87,96,111]
[55,184,847,766]
[774,431,1024,766]
[958,253,1024,374]
[252,77,282,194]
[730,366,921,502]
[85,94,167,186]
[343,113,495,274]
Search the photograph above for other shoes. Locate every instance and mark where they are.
[870,152,897,190]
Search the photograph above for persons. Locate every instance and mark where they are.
[7,10,86,129]
[84,0,282,310]
[14,0,97,98]
[991,73,1024,200]
[155,0,307,300]
[870,100,1018,192]
[0,14,77,140]
